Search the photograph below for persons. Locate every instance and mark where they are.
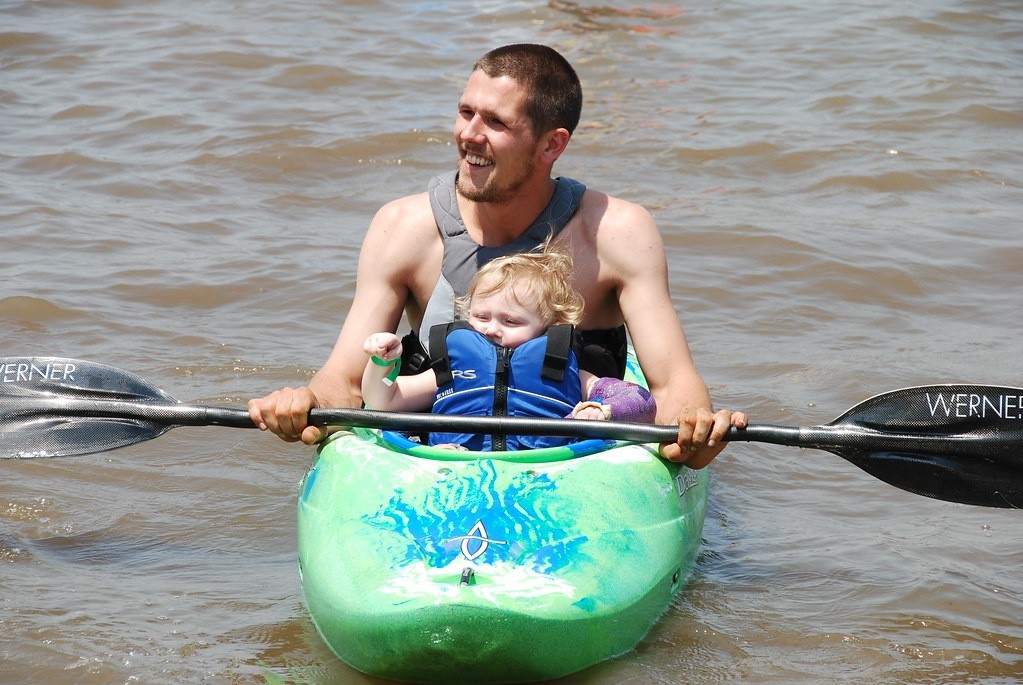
[362,254,656,451]
[248,43,749,469]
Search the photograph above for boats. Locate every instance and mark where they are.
[296,342,714,682]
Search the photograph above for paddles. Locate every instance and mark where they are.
[0,354,1022,512]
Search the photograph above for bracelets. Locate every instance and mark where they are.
[370,355,402,386]
